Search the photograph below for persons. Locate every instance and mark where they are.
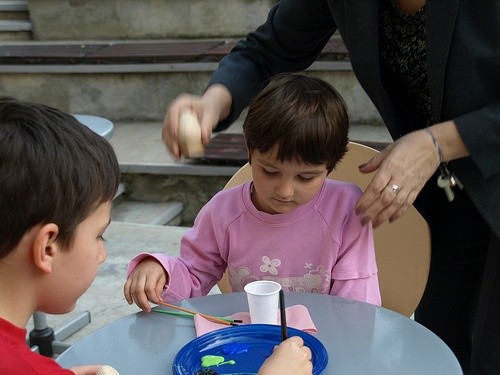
[123,73,383,312]
[162,0,500,375]
[0,95,314,375]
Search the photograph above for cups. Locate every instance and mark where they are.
[244,280,281,326]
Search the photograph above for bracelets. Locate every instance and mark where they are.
[424,128,443,164]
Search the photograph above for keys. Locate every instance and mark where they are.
[437,161,464,202]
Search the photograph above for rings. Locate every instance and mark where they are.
[392,184,398,190]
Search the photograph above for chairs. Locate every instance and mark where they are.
[216,140,432,318]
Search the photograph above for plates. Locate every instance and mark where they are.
[172,322,328,375]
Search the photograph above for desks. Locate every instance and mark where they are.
[54,293,463,375]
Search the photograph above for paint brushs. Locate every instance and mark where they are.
[156,300,238,326]
[279,289,289,341]
[151,308,243,323]
